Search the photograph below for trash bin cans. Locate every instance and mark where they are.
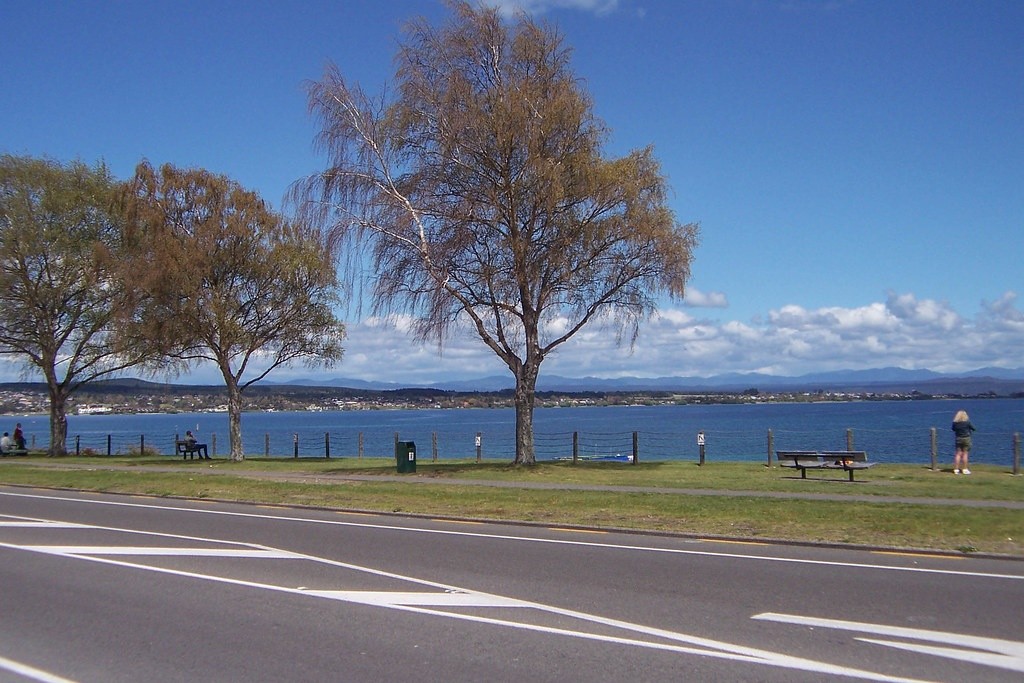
[397,441,416,473]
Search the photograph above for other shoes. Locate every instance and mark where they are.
[954,469,960,474]
[962,468,971,474]
[205,456,211,459]
[199,457,203,459]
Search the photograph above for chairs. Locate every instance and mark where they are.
[176,440,200,461]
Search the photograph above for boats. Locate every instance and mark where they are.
[552,454,635,462]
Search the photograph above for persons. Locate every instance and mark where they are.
[952,411,975,474]
[14,423,26,456]
[185,431,212,459]
[0,432,13,457]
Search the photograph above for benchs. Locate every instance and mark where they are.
[776,450,876,482]
